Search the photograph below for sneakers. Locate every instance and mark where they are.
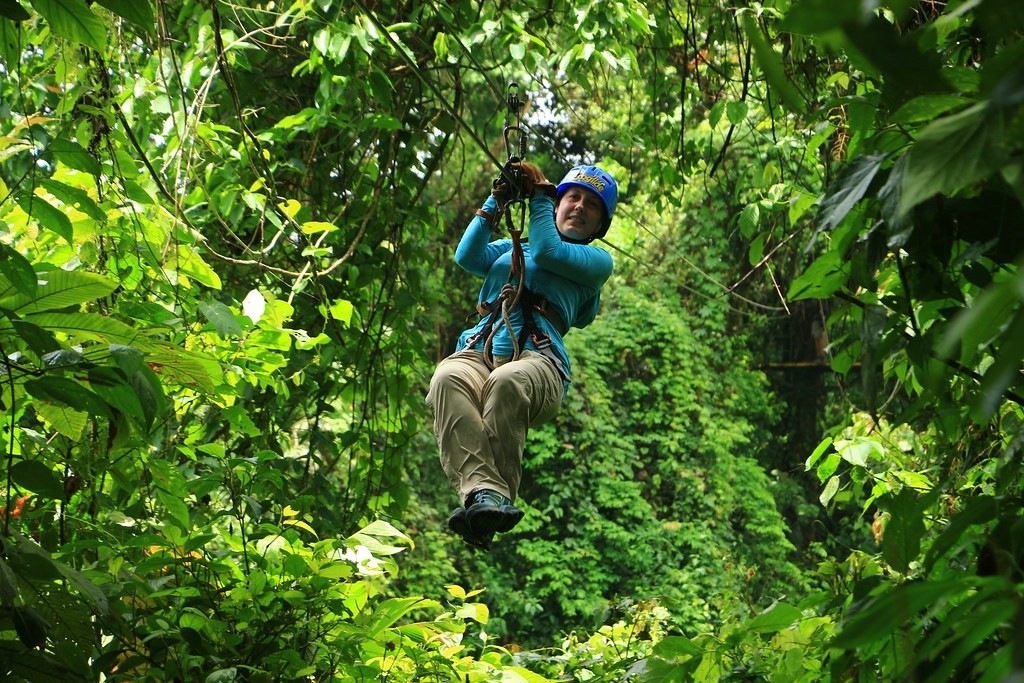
[448,490,525,550]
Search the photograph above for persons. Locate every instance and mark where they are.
[422,148,624,551]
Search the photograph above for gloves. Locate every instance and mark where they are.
[491,163,556,203]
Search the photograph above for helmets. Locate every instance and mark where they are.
[555,164,618,221]
